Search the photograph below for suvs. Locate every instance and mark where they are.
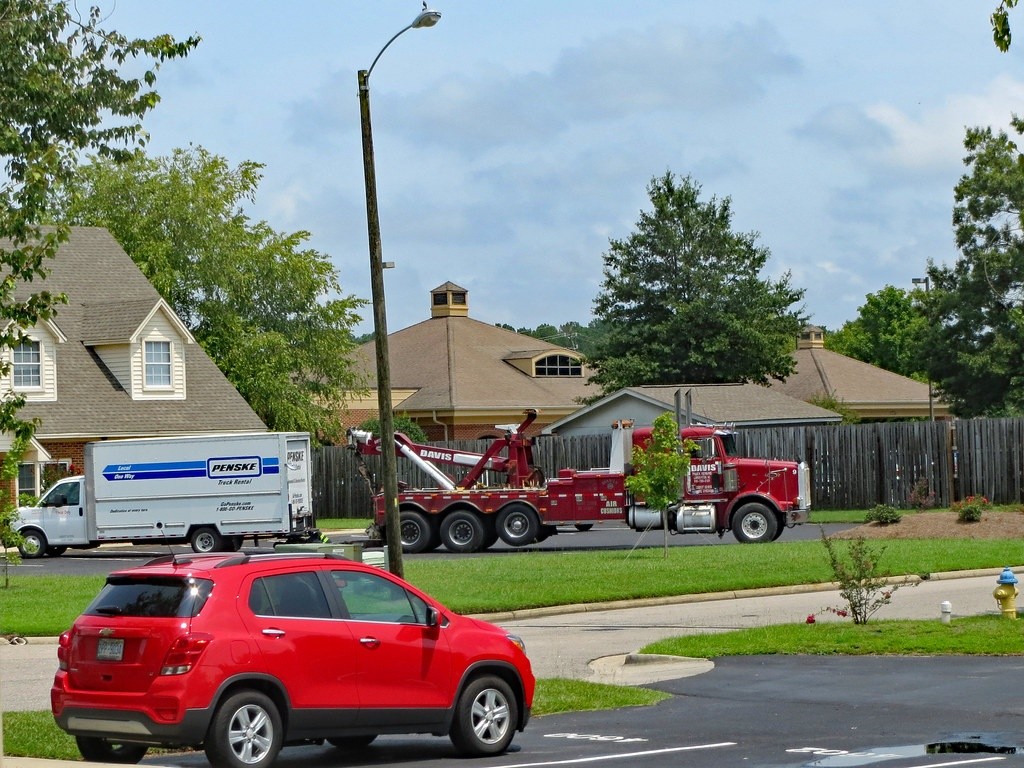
[51,547,537,768]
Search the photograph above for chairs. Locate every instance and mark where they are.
[251,582,320,617]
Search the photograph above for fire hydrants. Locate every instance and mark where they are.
[993,565,1019,621]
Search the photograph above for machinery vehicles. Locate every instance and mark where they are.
[345,388,811,554]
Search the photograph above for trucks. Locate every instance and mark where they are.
[9,432,319,560]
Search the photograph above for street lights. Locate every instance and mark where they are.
[357,8,442,603]
[911,277,933,421]
